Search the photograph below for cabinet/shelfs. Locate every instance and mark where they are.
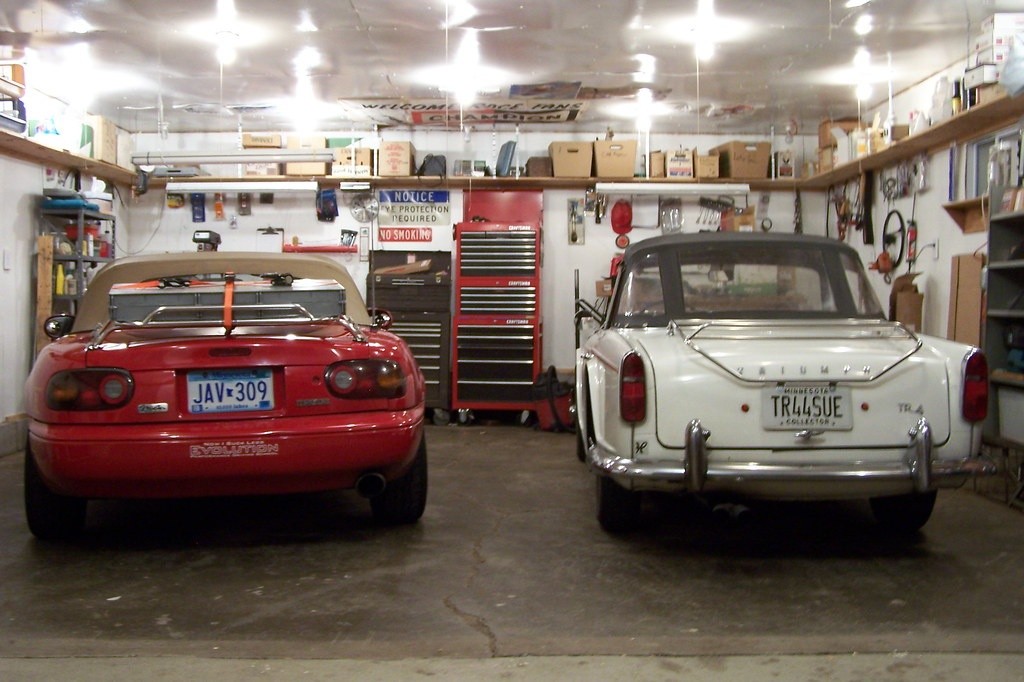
[36,208,117,315]
[981,128,1024,447]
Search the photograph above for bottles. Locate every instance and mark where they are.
[82,232,94,257]
[55,262,101,295]
[100,230,112,257]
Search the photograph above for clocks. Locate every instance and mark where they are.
[350,193,380,223]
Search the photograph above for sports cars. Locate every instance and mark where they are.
[566,230,993,542]
[18,251,428,541]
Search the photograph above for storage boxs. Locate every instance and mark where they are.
[0,12,1024,296]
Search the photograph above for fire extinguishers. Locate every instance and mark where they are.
[905,220,917,263]
